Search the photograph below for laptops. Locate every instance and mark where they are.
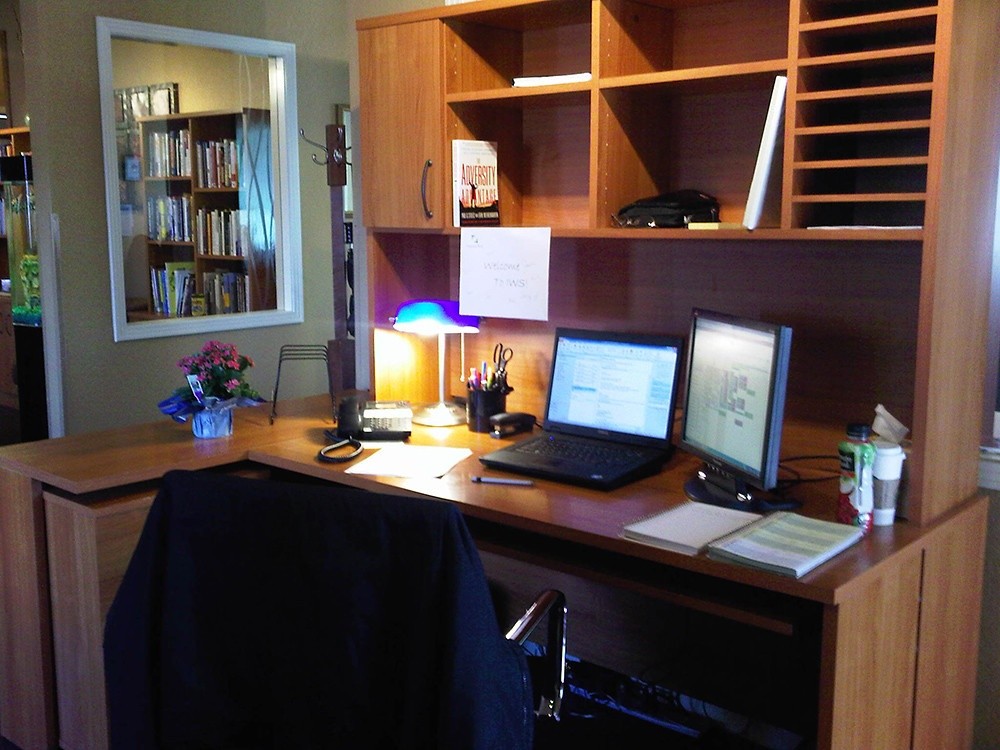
[480,327,685,492]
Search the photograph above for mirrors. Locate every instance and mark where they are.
[95,14,304,342]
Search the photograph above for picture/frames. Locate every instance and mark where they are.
[126,86,149,125]
[149,82,178,117]
[113,89,127,129]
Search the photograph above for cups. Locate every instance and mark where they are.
[873,440,904,527]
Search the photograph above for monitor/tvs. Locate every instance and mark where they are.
[678,308,804,514]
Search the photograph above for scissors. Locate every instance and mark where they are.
[492,343,513,371]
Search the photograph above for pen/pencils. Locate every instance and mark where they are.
[471,476,533,485]
[466,361,508,391]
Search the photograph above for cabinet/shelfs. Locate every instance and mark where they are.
[357,0,1000,524]
[0,127,31,408]
[127,107,277,322]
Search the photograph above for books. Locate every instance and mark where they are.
[621,500,761,557]
[146,127,251,318]
[709,510,864,580]
[743,74,789,230]
[513,72,592,90]
[451,139,500,228]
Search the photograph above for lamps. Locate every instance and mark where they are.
[393,299,480,427]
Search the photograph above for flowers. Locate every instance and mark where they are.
[159,340,259,401]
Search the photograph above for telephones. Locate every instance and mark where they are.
[339,400,411,440]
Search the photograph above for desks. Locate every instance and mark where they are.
[0,391,992,750]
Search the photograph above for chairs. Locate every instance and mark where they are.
[104,468,570,750]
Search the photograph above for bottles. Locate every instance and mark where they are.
[838,423,875,535]
[191,295,205,316]
